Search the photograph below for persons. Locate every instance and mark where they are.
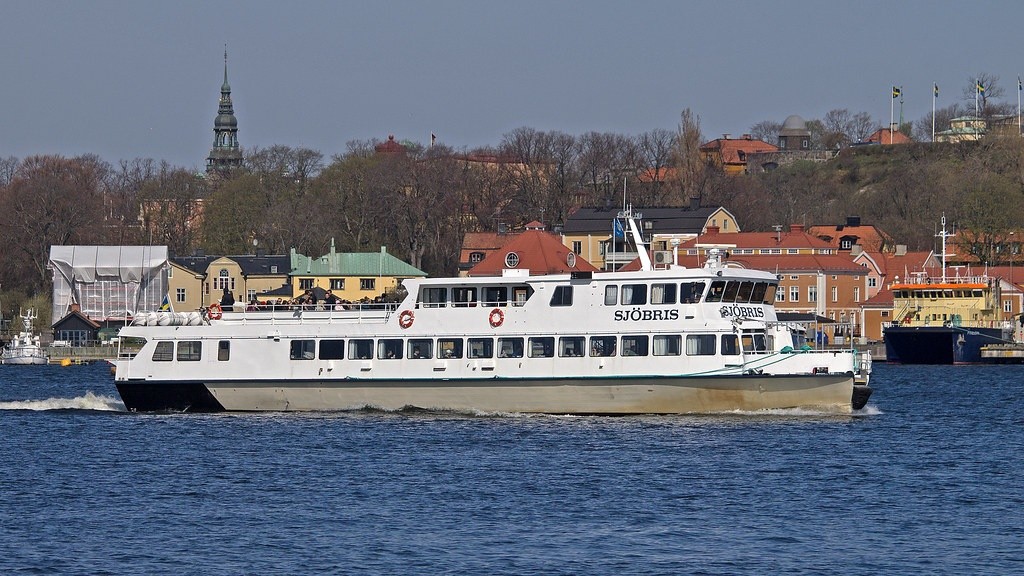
[565,347,580,357]
[444,348,459,358]
[501,346,522,357]
[628,345,640,355]
[544,345,552,356]
[473,349,480,357]
[413,348,426,359]
[251,288,400,309]
[591,345,616,356]
[386,349,396,359]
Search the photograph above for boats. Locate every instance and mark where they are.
[104,177,873,416]
[881,210,1016,365]
[0,306,49,365]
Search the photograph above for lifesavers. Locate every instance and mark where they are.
[489,308,504,327]
[208,304,222,320]
[399,309,414,329]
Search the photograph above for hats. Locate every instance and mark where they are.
[446,349,452,353]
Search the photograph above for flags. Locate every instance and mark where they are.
[893,87,900,98]
[935,83,938,97]
[1019,77,1022,90]
[978,81,985,98]
[615,220,624,238]
[156,295,172,312]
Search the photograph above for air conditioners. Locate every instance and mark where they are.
[654,251,672,265]
